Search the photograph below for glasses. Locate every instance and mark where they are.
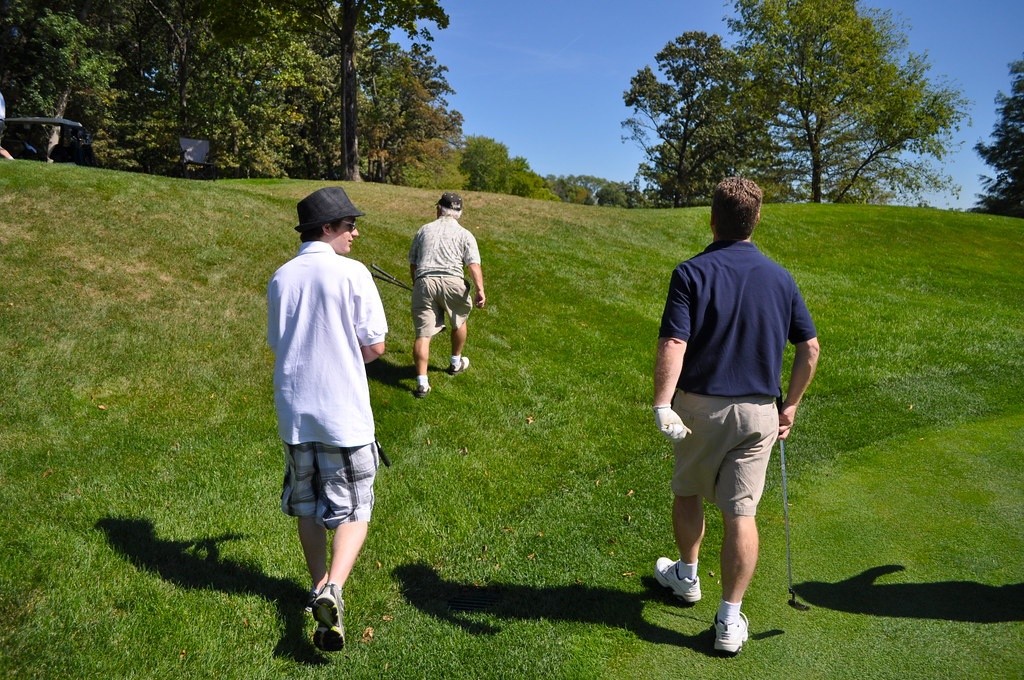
[340,220,356,231]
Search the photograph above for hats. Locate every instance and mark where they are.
[293,187,366,233]
[434,192,462,212]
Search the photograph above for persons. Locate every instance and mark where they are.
[409,193,486,398]
[268,186,389,652]
[650,178,820,654]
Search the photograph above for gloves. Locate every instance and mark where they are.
[651,405,692,440]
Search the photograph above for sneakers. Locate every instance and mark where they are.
[313,583,346,651]
[713,611,748,653]
[416,386,431,398]
[655,556,702,602]
[303,590,318,612]
[449,357,469,375]
